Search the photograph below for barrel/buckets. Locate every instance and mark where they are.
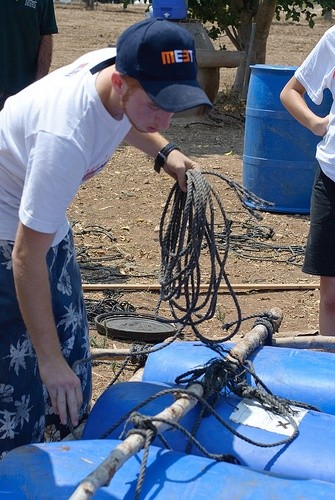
[152,0,188,20]
[82,381,334,484]
[0,438,334,500]
[143,343,335,416]
[242,64,334,214]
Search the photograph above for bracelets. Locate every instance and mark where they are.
[153,142,181,174]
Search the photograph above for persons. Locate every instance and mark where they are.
[278,25,335,334]
[1,0,60,113]
[1,15,213,457]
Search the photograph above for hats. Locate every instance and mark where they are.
[116,17,216,112]
